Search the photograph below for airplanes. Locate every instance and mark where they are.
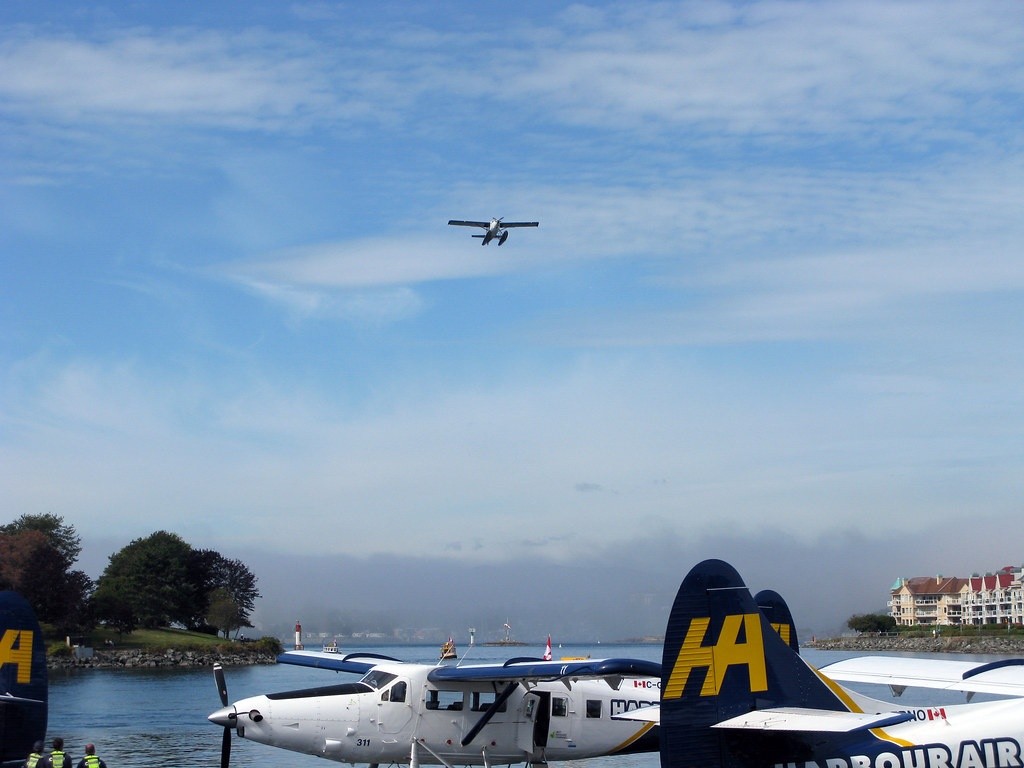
[447,214,541,245]
[206,555,1024,768]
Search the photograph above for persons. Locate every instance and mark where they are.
[76,743,107,768]
[43,738,73,768]
[240,634,244,645]
[22,741,45,768]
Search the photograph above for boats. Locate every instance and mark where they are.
[320,640,339,654]
[437,638,457,659]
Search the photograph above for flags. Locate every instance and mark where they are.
[543,636,552,662]
